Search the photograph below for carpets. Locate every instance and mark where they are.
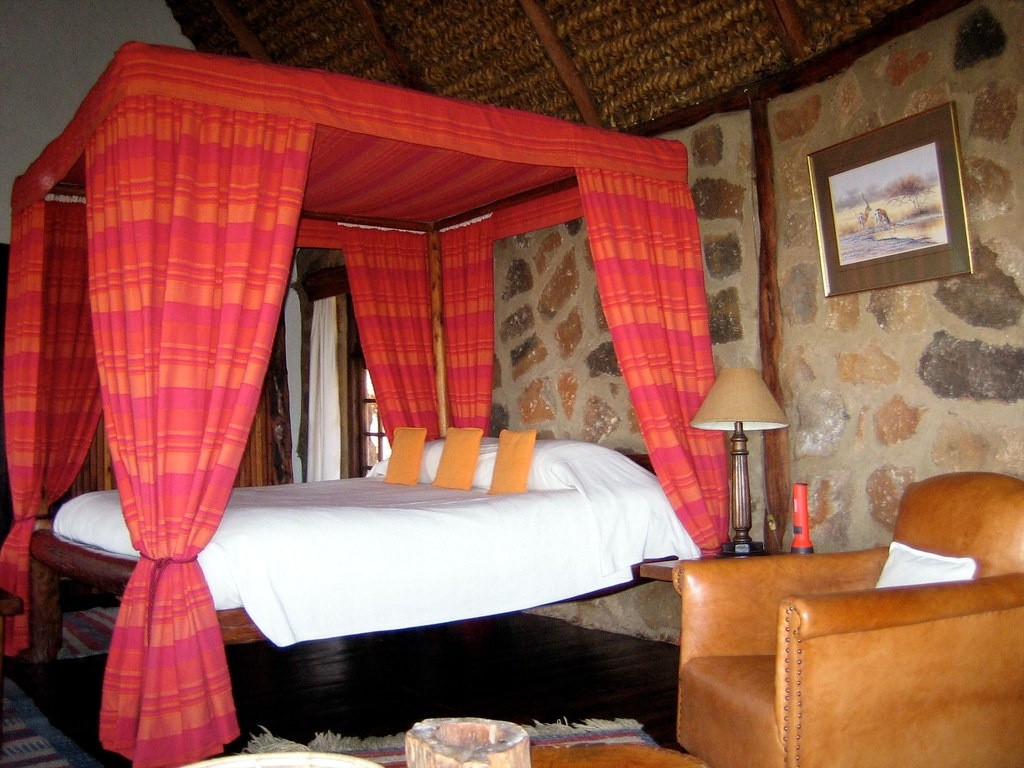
[0,675,103,768]
[56,607,125,659]
[244,714,707,768]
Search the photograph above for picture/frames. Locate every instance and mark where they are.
[805,100,975,298]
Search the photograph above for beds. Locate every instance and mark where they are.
[1,40,729,768]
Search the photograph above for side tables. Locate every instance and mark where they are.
[639,553,790,582]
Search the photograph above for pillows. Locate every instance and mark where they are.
[383,427,428,486]
[876,540,977,588]
[488,428,537,496]
[431,427,485,491]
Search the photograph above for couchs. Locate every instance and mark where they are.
[670,471,1024,768]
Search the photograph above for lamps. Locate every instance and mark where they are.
[690,366,793,555]
